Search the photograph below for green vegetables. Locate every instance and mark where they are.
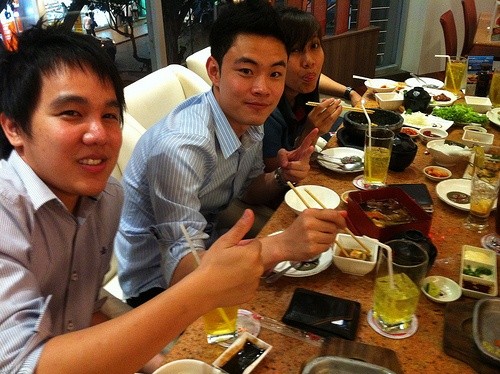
[462,265,492,279]
[405,108,412,115]
[396,90,408,94]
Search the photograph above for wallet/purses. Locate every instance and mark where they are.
[280,287,361,341]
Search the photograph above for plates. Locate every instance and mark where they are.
[474,298,500,361]
[301,357,398,374]
[267,230,332,277]
[485,108,500,126]
[459,245,498,299]
[423,88,457,107]
[211,332,273,374]
[341,189,360,204]
[435,179,497,211]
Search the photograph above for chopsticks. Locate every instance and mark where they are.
[287,181,371,258]
[306,102,375,114]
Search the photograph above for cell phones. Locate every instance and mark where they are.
[399,182,434,212]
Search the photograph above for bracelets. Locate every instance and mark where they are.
[275,167,297,187]
[343,87,354,99]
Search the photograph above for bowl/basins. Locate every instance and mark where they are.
[333,232,378,276]
[379,228,437,275]
[284,185,341,216]
[422,166,452,181]
[150,360,221,374]
[420,275,462,303]
[343,77,494,167]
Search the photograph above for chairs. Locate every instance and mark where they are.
[439,10,458,62]
[100,47,213,304]
[461,0,478,59]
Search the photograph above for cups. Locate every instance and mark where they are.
[373,239,428,332]
[192,249,237,344]
[444,56,500,108]
[460,145,500,254]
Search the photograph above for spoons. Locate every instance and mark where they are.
[311,151,364,171]
[264,254,321,284]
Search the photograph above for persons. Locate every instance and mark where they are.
[83,0,138,36]
[114,0,347,308]
[0,14,265,374]
[261,6,366,159]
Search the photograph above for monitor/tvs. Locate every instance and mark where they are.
[466,56,493,72]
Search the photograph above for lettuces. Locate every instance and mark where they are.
[433,103,490,124]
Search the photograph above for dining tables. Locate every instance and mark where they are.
[473,12,500,61]
[156,82,500,374]
